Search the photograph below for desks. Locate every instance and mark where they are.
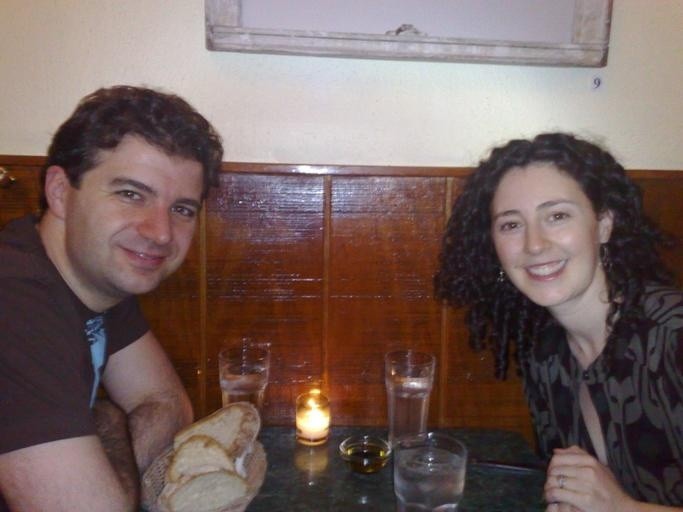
[194,417,548,512]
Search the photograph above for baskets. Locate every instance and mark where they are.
[140,440,268,511]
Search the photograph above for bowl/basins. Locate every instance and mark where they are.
[338,436,392,474]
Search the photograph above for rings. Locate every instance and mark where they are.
[559,475,566,488]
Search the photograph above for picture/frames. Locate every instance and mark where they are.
[204,0,613,68]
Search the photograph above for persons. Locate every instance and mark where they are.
[432,130,681,512]
[0,83,225,511]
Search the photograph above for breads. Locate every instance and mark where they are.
[156,400,261,512]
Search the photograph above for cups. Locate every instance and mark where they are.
[384,348,467,512]
[294,393,330,445]
[219,347,269,411]
